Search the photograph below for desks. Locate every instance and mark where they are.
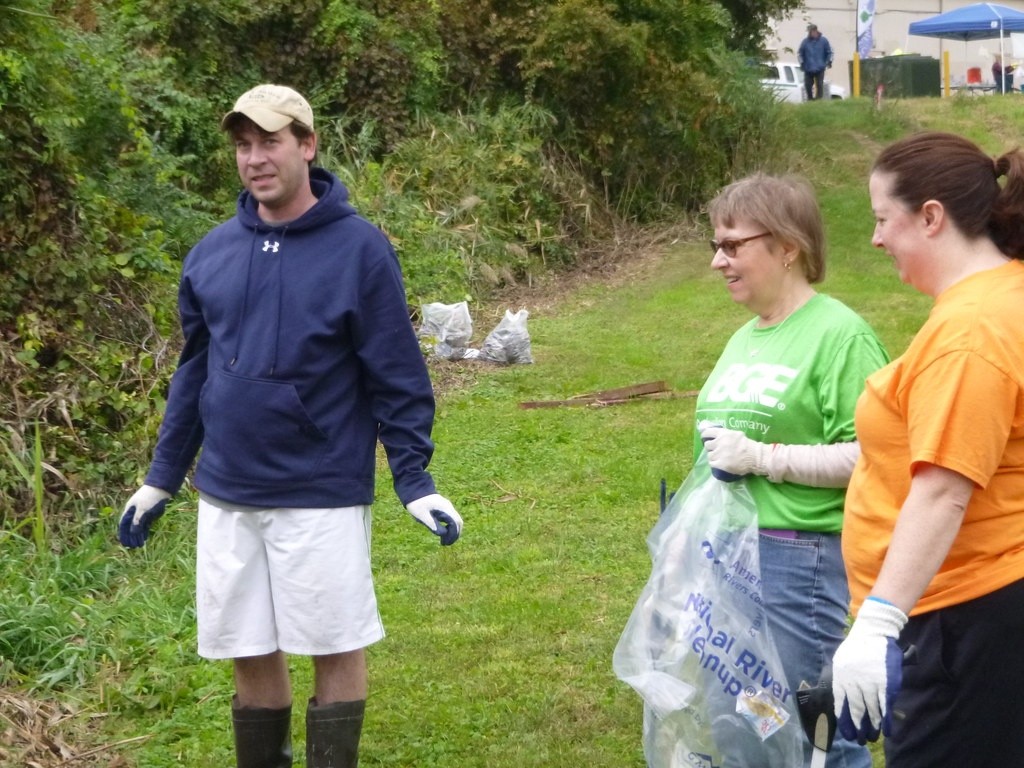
[949,85,1000,96]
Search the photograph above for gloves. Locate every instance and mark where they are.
[403,494,463,546]
[830,596,911,747]
[118,483,173,549]
[701,428,776,482]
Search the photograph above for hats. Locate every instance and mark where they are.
[219,83,316,133]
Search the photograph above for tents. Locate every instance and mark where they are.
[905,3,1024,95]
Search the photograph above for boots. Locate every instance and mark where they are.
[305,695,365,768]
[232,694,294,768]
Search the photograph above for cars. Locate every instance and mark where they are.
[760,62,846,103]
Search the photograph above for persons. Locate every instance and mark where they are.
[833,132,1023,767]
[992,54,1017,93]
[798,25,834,100]
[118,84,464,768]
[694,176,891,768]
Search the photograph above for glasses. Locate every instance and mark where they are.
[709,231,772,258]
